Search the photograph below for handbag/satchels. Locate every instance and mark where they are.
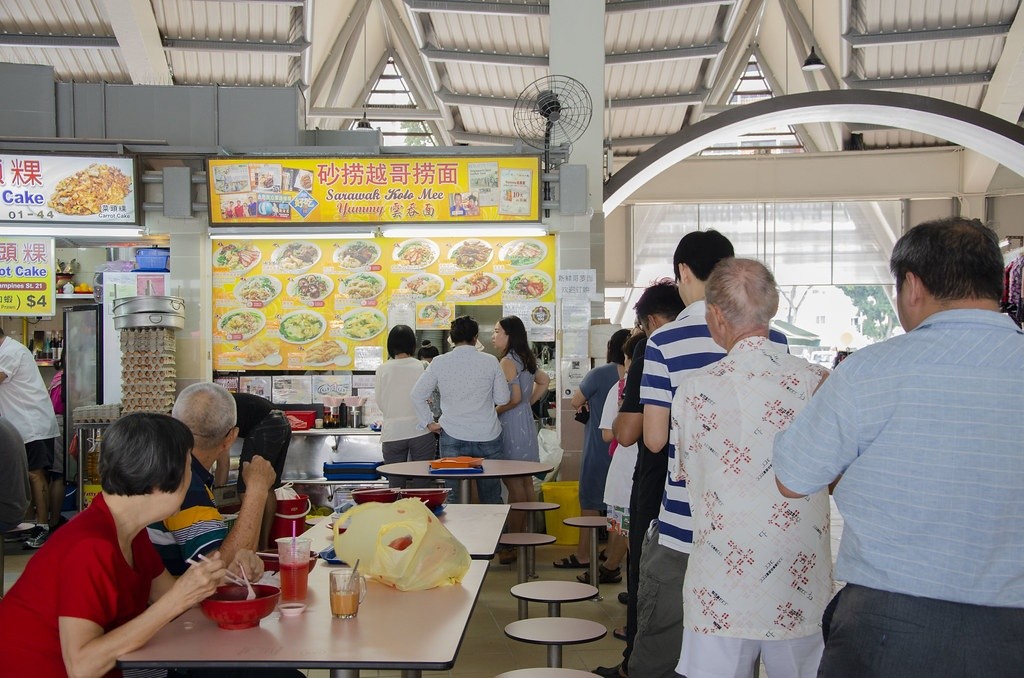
[332,497,473,593]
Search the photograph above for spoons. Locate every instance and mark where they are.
[238,562,256,600]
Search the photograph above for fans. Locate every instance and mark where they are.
[512,74,593,217]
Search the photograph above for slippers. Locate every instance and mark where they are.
[553,549,608,568]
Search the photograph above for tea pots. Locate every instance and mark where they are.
[63,281,74,294]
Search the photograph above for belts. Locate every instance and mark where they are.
[264,412,283,419]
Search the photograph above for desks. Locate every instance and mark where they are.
[116,558,490,678]
[298,503,512,678]
[377,460,555,504]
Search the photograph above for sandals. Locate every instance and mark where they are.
[577,563,622,584]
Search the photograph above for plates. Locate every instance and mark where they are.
[213,238,553,366]
[279,603,306,615]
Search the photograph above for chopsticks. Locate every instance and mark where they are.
[187,554,245,586]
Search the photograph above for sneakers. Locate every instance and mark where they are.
[23,526,50,549]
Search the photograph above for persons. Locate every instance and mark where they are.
[372,316,550,566]
[0,328,70,549]
[214,392,292,552]
[551,217,1024,678]
[146,382,305,678]
[0,412,264,678]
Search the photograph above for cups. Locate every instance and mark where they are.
[349,406,362,428]
[275,537,312,602]
[330,569,367,619]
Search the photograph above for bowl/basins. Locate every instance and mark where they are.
[351,489,399,505]
[262,550,319,575]
[199,584,280,630]
[401,489,448,511]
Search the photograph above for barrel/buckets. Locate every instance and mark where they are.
[270,494,311,549]
[541,481,580,545]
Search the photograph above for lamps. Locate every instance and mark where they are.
[0,226,148,237]
[208,226,379,239]
[354,17,373,130]
[380,223,550,238]
[801,0,826,72]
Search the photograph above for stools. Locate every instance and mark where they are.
[510,580,599,617]
[496,668,603,678]
[504,617,607,668]
[498,533,556,621]
[510,501,561,578]
[563,517,608,602]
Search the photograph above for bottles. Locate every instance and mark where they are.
[56,273,75,294]
[27,329,64,360]
[83,428,103,484]
[315,399,347,429]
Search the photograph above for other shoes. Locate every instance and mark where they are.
[4,530,25,542]
[613,626,627,641]
[499,548,517,564]
[591,663,626,678]
[618,592,627,605]
